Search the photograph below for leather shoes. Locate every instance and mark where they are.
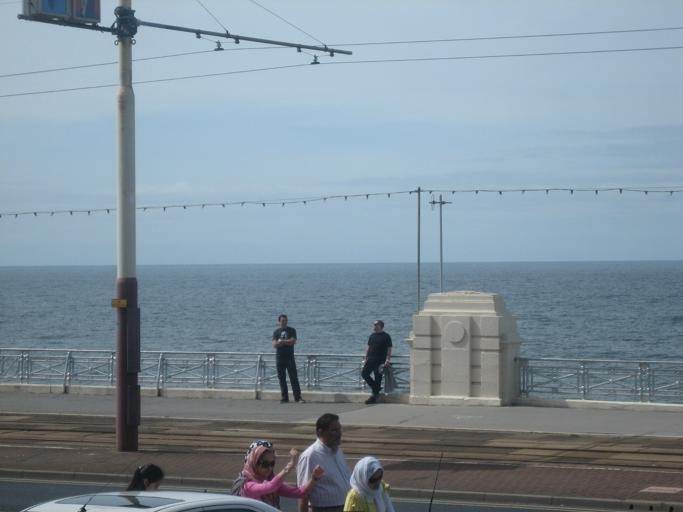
[298,398,305,403]
[279,398,288,402]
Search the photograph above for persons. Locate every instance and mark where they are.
[343,455,395,512]
[231,440,325,509]
[296,413,353,512]
[361,320,392,404]
[272,314,305,403]
[127,464,164,491]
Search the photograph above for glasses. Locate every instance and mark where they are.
[370,475,382,483]
[259,461,275,468]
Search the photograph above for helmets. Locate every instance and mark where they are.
[373,320,385,328]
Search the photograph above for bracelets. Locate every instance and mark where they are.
[312,475,321,480]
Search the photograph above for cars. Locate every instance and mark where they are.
[19,490,283,512]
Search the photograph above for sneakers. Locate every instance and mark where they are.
[374,386,380,394]
[365,397,375,404]
